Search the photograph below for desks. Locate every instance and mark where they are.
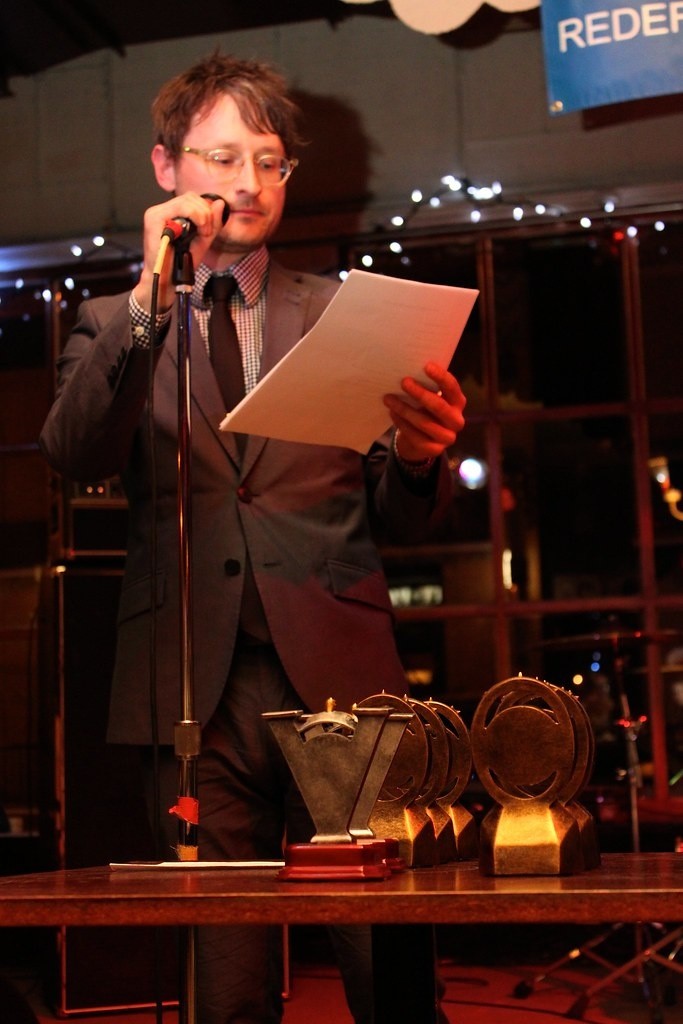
[0,851,683,1024]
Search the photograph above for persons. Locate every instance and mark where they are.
[41,56,467,1024]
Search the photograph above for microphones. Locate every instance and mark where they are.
[161,193,230,244]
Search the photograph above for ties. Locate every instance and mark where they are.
[206,272,249,463]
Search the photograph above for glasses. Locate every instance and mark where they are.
[178,146,300,186]
